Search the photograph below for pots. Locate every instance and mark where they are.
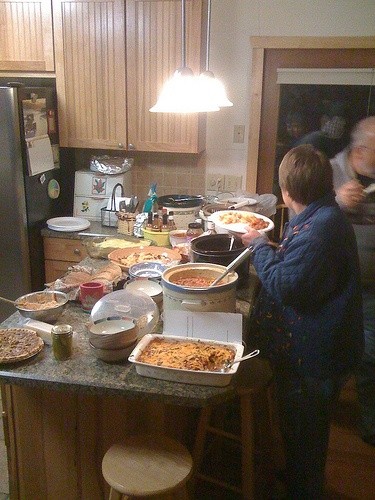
[161,262,239,316]
[156,195,203,231]
[190,234,250,285]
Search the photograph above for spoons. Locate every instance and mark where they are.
[214,349,260,373]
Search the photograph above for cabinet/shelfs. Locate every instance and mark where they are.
[44,238,88,289]
[0,0,206,154]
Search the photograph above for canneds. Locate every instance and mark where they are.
[51,325,73,360]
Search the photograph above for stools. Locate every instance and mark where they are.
[190,355,284,500]
[102,434,194,500]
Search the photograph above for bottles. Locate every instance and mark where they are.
[146,206,176,232]
[185,218,217,262]
[51,324,74,361]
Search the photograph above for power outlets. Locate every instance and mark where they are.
[207,173,224,192]
[224,175,242,193]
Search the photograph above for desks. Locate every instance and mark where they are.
[0,256,262,500]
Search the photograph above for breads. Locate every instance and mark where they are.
[64,264,122,287]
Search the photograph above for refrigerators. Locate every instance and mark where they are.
[0,77,75,324]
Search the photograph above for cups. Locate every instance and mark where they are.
[80,282,104,310]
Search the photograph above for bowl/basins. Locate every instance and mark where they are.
[14,291,69,323]
[199,204,275,241]
[168,230,186,245]
[80,236,154,259]
[122,261,167,303]
[108,246,183,272]
[141,225,178,246]
[86,316,139,363]
[172,243,193,264]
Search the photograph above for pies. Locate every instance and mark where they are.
[0,328,44,363]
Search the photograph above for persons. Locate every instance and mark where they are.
[322,116,375,450]
[241,143,358,500]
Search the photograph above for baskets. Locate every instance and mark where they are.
[116,211,136,235]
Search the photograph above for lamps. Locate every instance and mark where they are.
[198,0,233,107]
[149,0,220,113]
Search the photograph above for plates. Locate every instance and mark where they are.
[46,217,91,232]
[1,328,45,364]
